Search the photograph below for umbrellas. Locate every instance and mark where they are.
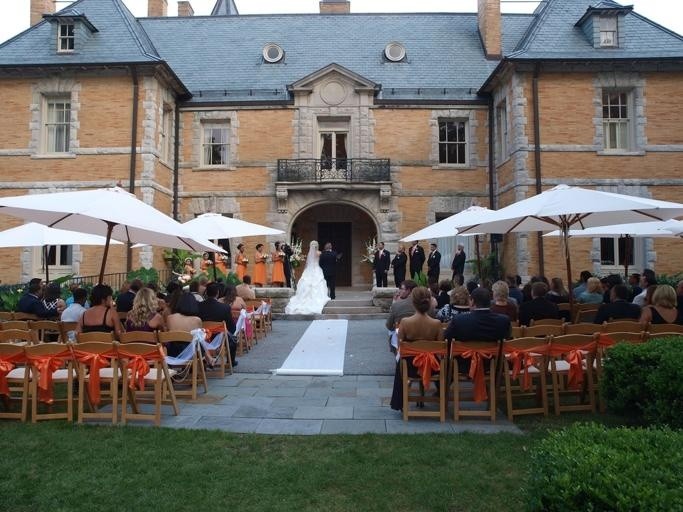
[128,214,285,256]
[539,220,683,280]
[0,222,123,282]
[399,207,497,279]
[454,184,683,289]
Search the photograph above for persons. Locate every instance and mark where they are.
[81,285,123,342]
[643,286,658,307]
[118,279,143,312]
[391,245,407,287]
[600,274,622,303]
[451,244,466,285]
[157,280,184,300]
[252,244,267,287]
[14,283,66,319]
[467,281,478,298]
[639,284,683,324]
[437,274,470,321]
[184,257,197,275]
[285,240,331,314]
[594,285,641,324]
[676,280,683,297]
[372,242,391,287]
[189,275,211,302]
[390,286,444,412]
[572,271,592,298]
[66,284,90,310]
[234,244,249,281]
[446,288,525,374]
[504,275,523,299]
[490,280,518,322]
[438,279,453,307]
[318,242,337,300]
[148,280,167,300]
[201,252,213,278]
[200,282,238,367]
[409,241,425,280]
[125,287,164,344]
[522,284,533,302]
[218,285,248,310]
[281,242,297,290]
[235,275,256,300]
[427,243,441,284]
[115,281,131,310]
[271,241,285,287]
[41,284,64,332]
[385,280,417,355]
[58,288,86,341]
[628,273,643,297]
[518,281,560,326]
[575,277,603,323]
[167,291,202,331]
[631,269,660,298]
[475,280,490,307]
[516,275,522,290]
[215,246,229,277]
[29,278,44,289]
[547,278,570,302]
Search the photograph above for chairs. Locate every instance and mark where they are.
[69,340,119,424]
[259,297,273,332]
[449,338,503,423]
[549,334,600,418]
[247,305,259,347]
[229,307,251,359]
[251,300,268,340]
[0,343,30,421]
[25,339,74,423]
[117,336,179,427]
[202,320,234,379]
[1,309,156,343]
[503,337,548,422]
[394,303,682,337]
[596,332,647,413]
[157,327,208,401]
[398,339,449,424]
